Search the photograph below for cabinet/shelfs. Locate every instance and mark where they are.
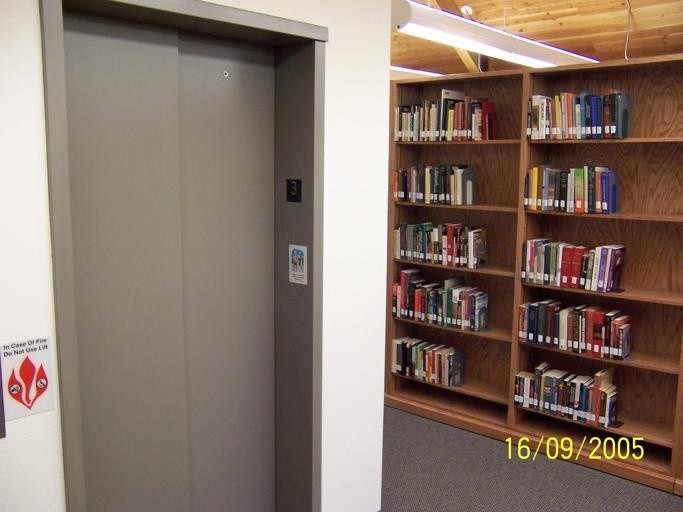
[385,52,683,497]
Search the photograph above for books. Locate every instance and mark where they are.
[391,338,464,388]
[514,361,618,428]
[393,163,476,206]
[394,89,496,141]
[522,238,627,293]
[518,300,631,360]
[524,164,618,214]
[392,268,489,332]
[392,222,488,270]
[526,92,631,141]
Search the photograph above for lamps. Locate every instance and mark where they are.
[392,0,599,68]
